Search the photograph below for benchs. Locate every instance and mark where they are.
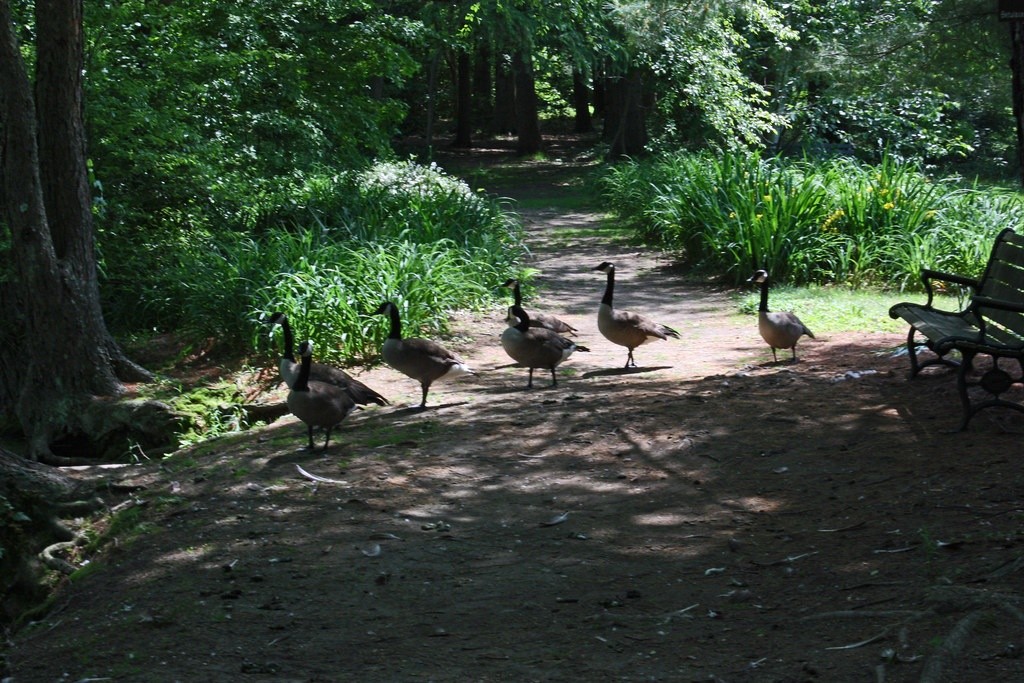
[887,228,1024,430]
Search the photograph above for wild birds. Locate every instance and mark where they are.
[368,301,482,412]
[499,278,590,389]
[746,268,816,366]
[261,311,390,456]
[590,261,680,373]
